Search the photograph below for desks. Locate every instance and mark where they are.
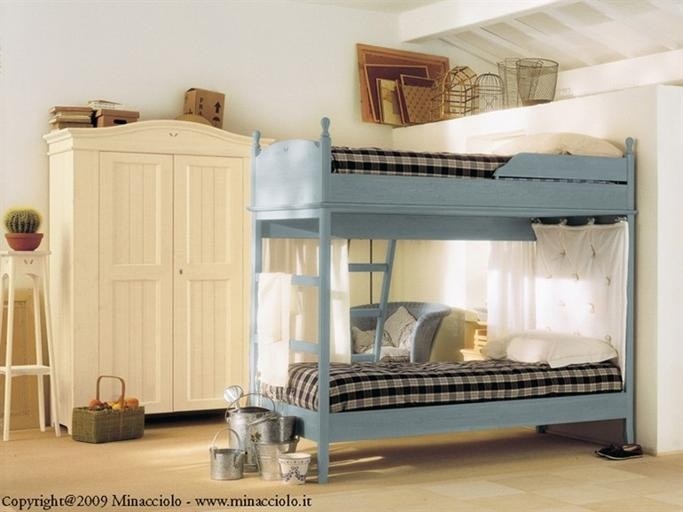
[1,248,60,442]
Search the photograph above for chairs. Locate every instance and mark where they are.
[350,298,452,369]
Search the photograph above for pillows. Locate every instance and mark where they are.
[523,128,625,158]
[472,328,619,367]
[351,305,418,363]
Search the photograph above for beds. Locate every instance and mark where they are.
[239,115,642,486]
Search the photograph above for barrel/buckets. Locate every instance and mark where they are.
[225,392,280,473]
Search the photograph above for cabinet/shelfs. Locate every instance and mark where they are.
[43,117,277,433]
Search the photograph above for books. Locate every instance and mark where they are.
[49,106,95,130]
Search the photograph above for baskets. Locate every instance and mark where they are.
[72,376,144,443]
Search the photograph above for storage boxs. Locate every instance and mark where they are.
[94,108,140,128]
[186,85,227,129]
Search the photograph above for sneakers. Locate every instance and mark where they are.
[597,443,644,460]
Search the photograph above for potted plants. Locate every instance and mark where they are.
[2,206,43,249]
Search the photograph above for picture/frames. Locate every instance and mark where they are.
[354,41,451,126]
